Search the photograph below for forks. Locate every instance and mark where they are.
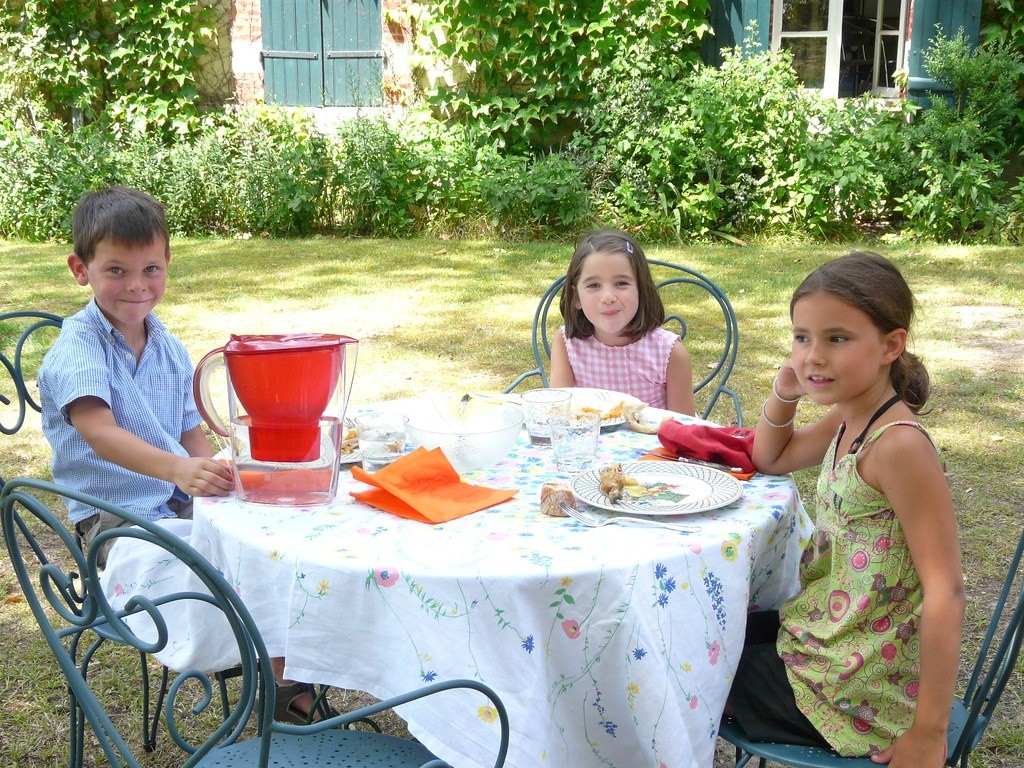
[557,500,703,534]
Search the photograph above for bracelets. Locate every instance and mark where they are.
[774,377,803,404]
[762,400,796,429]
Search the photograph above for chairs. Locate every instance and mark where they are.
[716,524,1022,768]
[497,255,744,438]
[0,477,508,768]
[0,310,344,768]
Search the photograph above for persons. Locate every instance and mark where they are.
[720,251,967,768]
[38,190,328,728]
[548,231,697,418]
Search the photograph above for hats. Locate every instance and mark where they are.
[658,420,756,472]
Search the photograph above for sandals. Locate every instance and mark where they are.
[253,680,355,730]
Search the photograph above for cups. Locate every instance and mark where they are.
[545,411,601,477]
[520,388,573,446]
[353,413,409,474]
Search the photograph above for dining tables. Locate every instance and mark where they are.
[101,388,813,766]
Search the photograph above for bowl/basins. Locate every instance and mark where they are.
[403,401,524,473]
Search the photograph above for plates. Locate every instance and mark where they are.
[569,460,743,517]
[338,447,364,467]
[548,387,643,430]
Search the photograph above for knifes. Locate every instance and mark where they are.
[631,446,742,474]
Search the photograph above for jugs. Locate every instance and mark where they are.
[187,327,359,510]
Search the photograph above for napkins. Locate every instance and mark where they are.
[350,443,520,524]
[641,414,761,480]
